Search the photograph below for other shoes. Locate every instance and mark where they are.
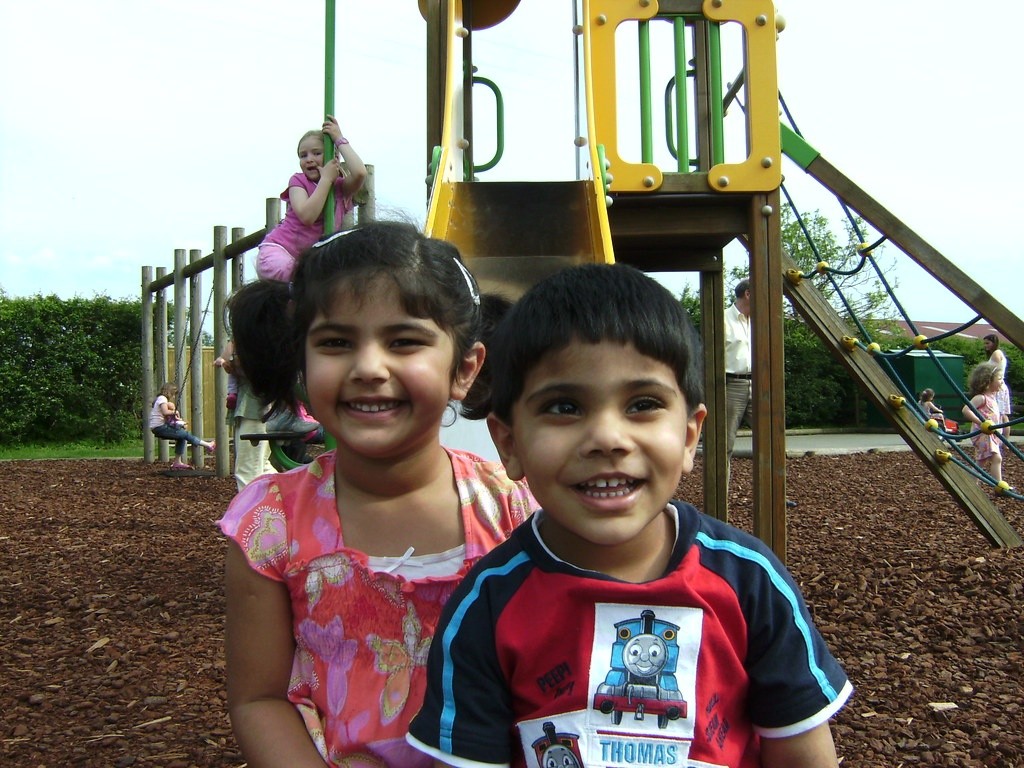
[785,498,797,506]
[227,394,237,409]
[208,439,217,451]
[170,461,191,468]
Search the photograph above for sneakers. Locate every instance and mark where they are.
[266,403,319,433]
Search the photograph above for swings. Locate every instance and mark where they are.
[149,266,217,441]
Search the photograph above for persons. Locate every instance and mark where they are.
[917,388,953,433]
[725,278,798,509]
[403,265,852,768]
[214,112,367,495]
[961,334,1011,491]
[149,381,214,469]
[217,210,542,768]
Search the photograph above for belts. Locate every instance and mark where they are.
[726,372,752,380]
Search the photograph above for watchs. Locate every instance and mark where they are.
[334,137,349,148]
